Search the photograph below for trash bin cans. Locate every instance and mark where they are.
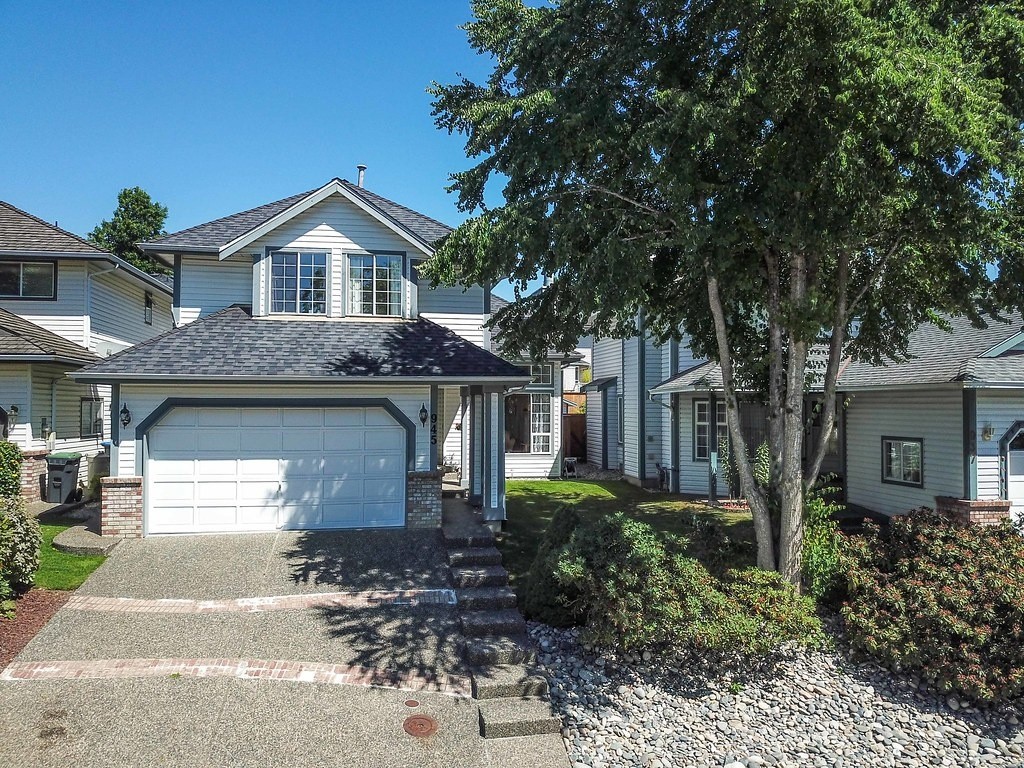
[45,451,82,503]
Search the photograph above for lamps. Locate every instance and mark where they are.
[419,403,428,427]
[983,420,995,441]
[120,402,131,428]
